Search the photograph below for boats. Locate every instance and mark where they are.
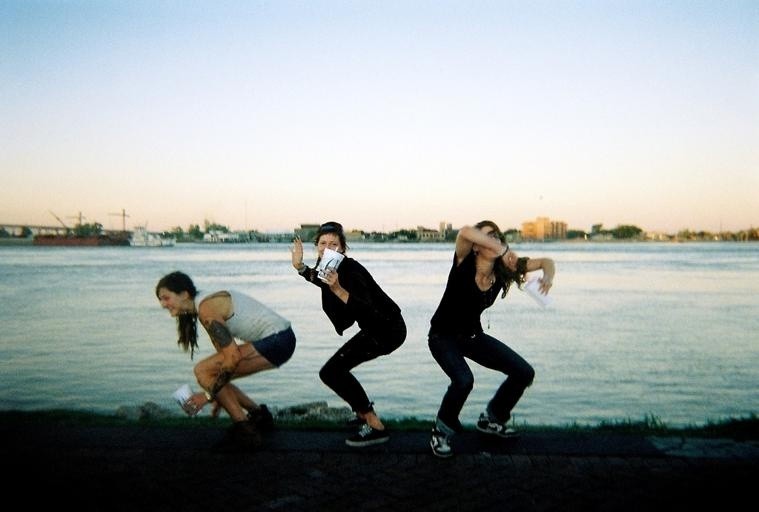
[32,230,134,246]
[129,226,176,248]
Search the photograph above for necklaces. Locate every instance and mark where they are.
[478,270,495,286]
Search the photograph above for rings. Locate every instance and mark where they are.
[186,399,197,414]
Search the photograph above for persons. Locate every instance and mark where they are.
[427,220,556,460]
[286,221,409,449]
[153,269,297,454]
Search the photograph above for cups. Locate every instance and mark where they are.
[317,249,345,281]
[522,277,553,309]
[172,382,202,415]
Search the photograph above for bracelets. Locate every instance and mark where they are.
[498,245,509,258]
[203,389,214,402]
[335,288,345,296]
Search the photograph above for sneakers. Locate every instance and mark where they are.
[247,403,274,431]
[429,422,453,458]
[345,423,390,448]
[475,411,518,438]
